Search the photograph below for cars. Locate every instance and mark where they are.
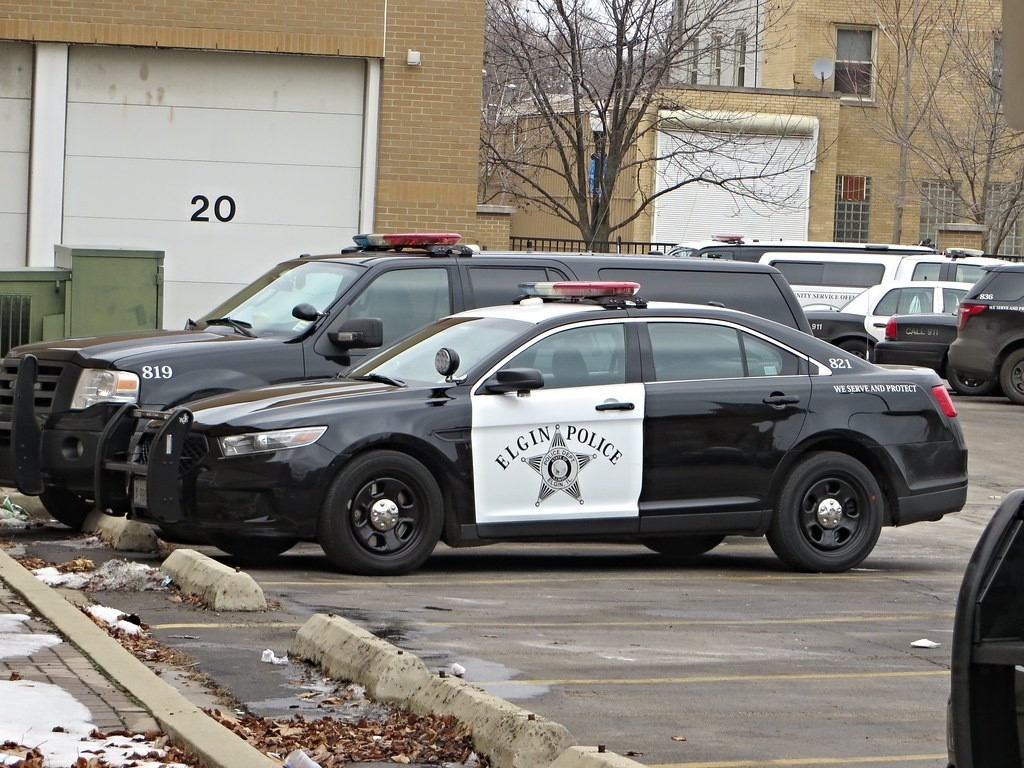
[803,281,975,375]
[874,312,1000,396]
[93,282,970,574]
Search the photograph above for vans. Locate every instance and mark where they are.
[647,233,939,264]
[1,231,815,535]
[755,247,1015,365]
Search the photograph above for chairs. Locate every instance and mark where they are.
[549,347,591,386]
[363,286,413,342]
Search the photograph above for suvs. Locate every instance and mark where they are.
[947,263,1024,406]
